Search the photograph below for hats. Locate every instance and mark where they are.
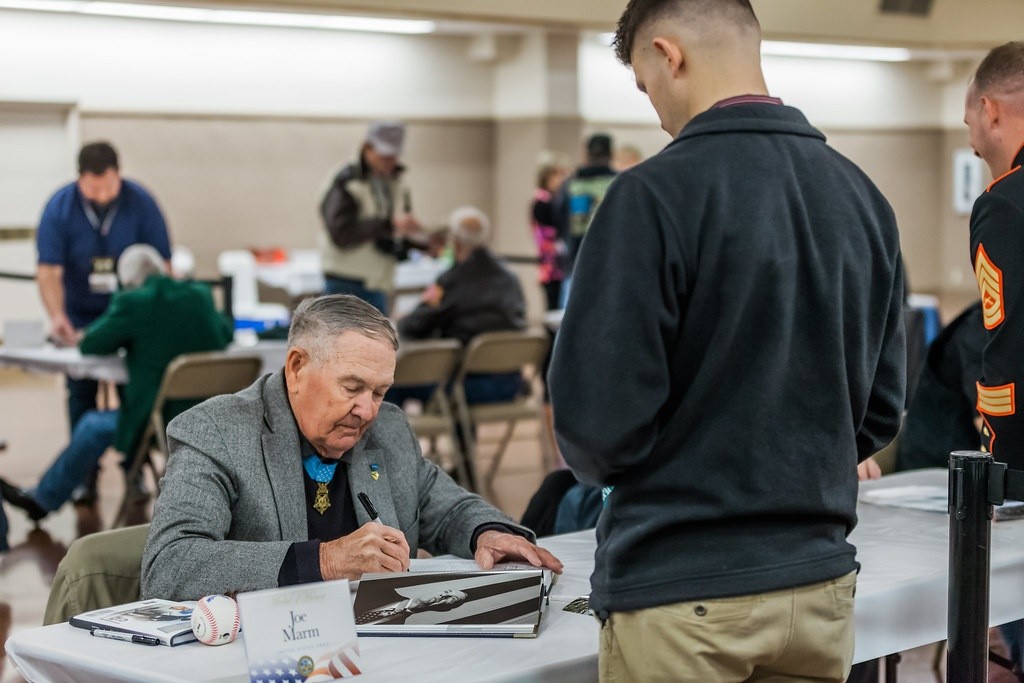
[368,122,405,158]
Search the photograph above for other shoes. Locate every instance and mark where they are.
[0,479,48,520]
[69,465,98,507]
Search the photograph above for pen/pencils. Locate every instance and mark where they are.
[356,491,416,573]
[90,627,159,645]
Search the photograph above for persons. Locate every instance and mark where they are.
[140,295,563,601]
[895,299,1024,683]
[530,132,642,309]
[37,142,173,528]
[547,0,906,683]
[318,124,445,318]
[383,207,526,409]
[0,244,234,521]
[965,41,1024,470]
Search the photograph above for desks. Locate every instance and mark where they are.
[0,327,399,413]
[4,469,1024,683]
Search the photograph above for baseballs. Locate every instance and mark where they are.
[191,593,241,647]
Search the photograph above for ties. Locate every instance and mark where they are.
[356,608,406,625]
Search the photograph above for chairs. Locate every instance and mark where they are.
[43,332,574,628]
